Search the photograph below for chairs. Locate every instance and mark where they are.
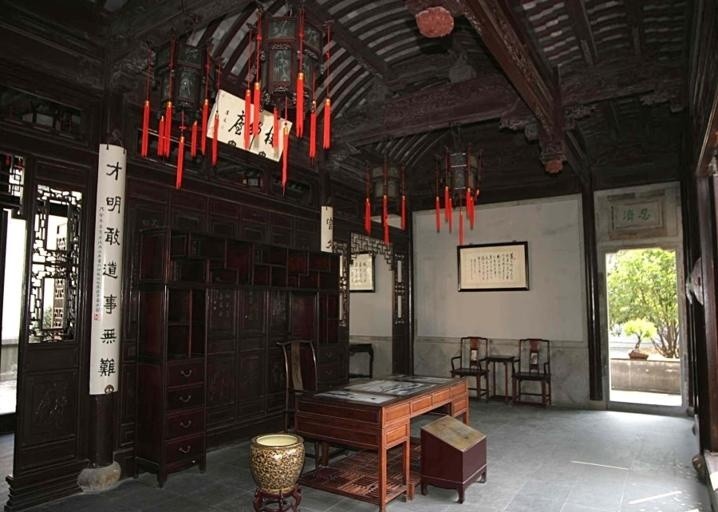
[449,336,488,403]
[511,338,551,407]
[348,342,375,381]
[274,337,347,472]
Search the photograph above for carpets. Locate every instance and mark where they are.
[297,436,421,506]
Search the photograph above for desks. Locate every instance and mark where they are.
[294,375,471,512]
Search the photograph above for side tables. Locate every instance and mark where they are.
[487,354,516,402]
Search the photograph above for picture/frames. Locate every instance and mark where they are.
[457,241,529,292]
[349,250,377,294]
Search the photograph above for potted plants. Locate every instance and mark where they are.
[623,317,657,359]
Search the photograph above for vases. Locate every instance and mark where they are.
[248,432,306,503]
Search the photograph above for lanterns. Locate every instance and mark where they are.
[139,32,227,191]
[432,122,483,248]
[242,1,336,200]
[360,150,409,249]
